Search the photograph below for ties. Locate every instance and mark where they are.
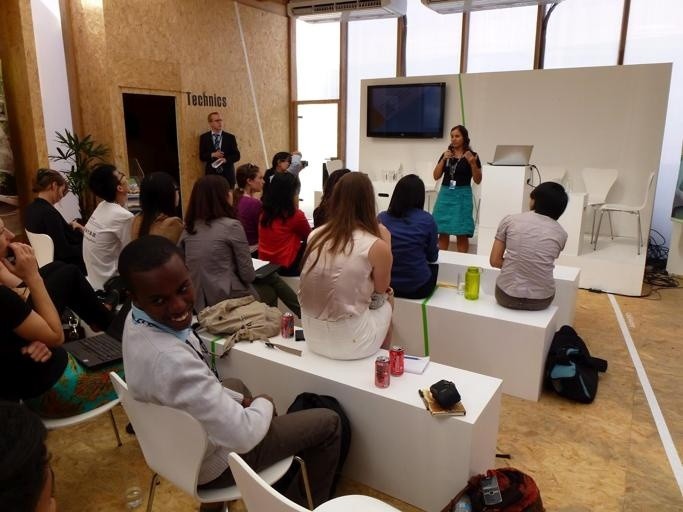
[213,134,223,174]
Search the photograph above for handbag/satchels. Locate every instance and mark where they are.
[543,325,607,404]
[191,295,283,357]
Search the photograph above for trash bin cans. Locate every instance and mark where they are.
[665,205,683,276]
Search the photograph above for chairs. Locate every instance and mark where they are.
[228,449,399,512]
[21,227,305,448]
[111,372,313,510]
[324,158,620,254]
[594,172,656,255]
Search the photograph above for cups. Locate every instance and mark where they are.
[457,272,465,295]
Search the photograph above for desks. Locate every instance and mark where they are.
[186,313,504,512]
[278,277,559,403]
[433,250,582,332]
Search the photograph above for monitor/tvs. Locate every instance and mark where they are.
[367,82,446,138]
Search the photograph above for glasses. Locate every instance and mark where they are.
[247,161,255,168]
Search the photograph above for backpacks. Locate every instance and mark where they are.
[272,391,352,510]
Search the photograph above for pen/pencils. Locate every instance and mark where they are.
[404,356,421,360]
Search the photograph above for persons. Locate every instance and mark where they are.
[0,403,57,512]
[491,181,570,310]
[0,221,123,422]
[198,113,242,189]
[433,125,481,253]
[120,234,351,511]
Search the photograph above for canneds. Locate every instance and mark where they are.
[389,346,404,376]
[282,312,294,338]
[375,356,390,388]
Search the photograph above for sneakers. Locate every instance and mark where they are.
[96,287,120,333]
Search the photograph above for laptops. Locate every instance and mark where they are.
[65,298,131,368]
[487,145,534,165]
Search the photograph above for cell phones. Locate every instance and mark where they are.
[480,475,503,506]
[295,330,305,341]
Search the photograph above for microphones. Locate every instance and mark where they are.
[446,146,453,168]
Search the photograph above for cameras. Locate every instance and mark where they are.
[288,156,308,167]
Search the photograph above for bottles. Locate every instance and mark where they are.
[210,158,223,169]
[465,267,480,301]
[454,493,472,512]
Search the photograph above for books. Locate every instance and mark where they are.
[418,387,468,418]
